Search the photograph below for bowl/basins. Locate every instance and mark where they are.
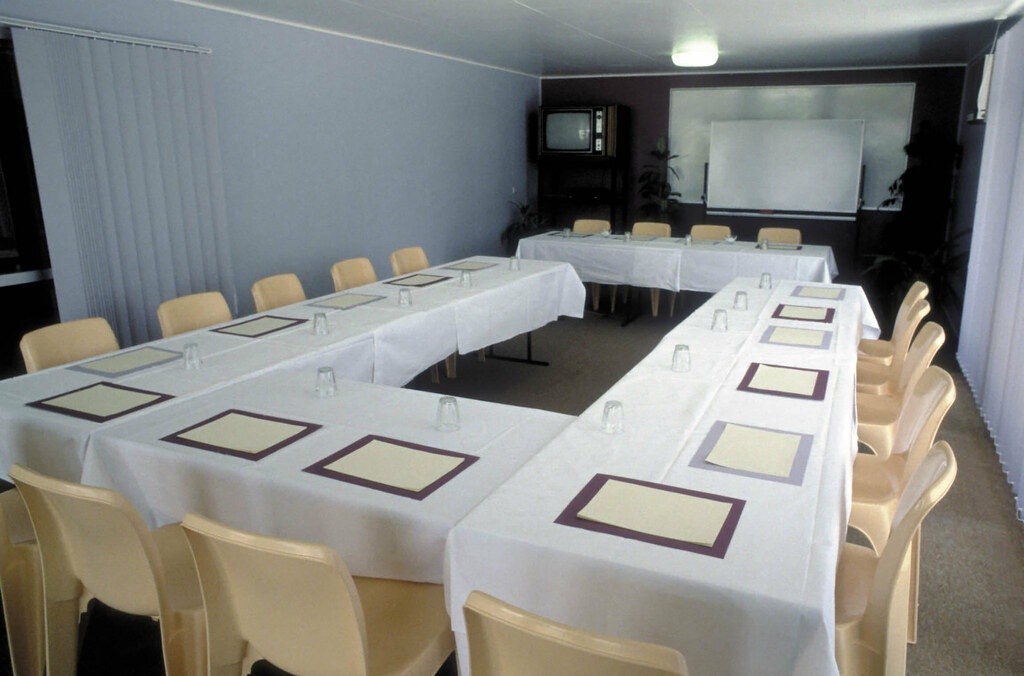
[599,229,612,237]
[723,235,737,243]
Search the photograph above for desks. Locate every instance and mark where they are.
[0,229,881,676]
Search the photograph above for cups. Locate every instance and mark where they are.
[758,273,773,290]
[733,291,748,311]
[398,288,413,308]
[671,345,691,371]
[509,255,520,271]
[711,309,727,331]
[624,232,631,244]
[313,313,329,335]
[315,366,337,396]
[684,234,693,246]
[460,271,474,287]
[601,400,624,434]
[760,239,769,251]
[562,228,571,241]
[434,396,462,430]
[182,341,202,369]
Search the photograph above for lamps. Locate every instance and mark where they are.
[672,50,719,67]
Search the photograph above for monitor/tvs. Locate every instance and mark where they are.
[537,104,617,158]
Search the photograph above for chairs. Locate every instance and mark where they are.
[181,510,455,676]
[390,246,485,379]
[610,223,671,318]
[20,317,120,374]
[847,365,957,644]
[758,228,801,244]
[6,460,208,676]
[330,257,441,386]
[856,298,932,396]
[0,487,93,676]
[834,440,959,676]
[251,273,308,312]
[857,280,929,365]
[856,321,947,456]
[573,219,611,311]
[462,590,688,676]
[670,225,732,317]
[157,291,233,338]
